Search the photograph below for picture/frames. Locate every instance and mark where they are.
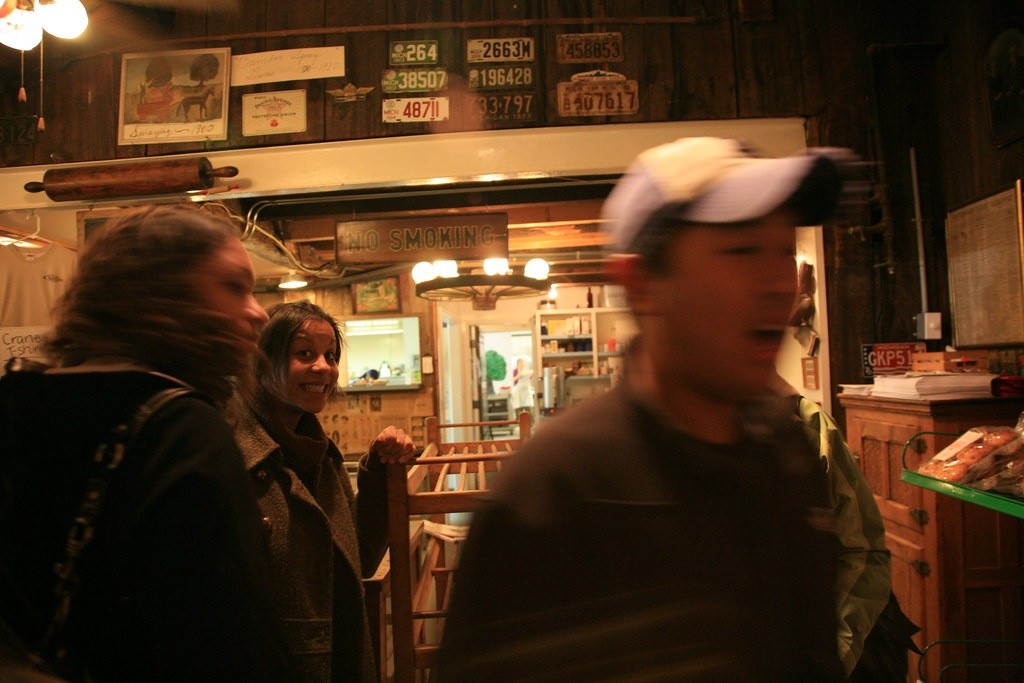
[349,274,404,315]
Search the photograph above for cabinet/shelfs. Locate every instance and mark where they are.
[384,411,531,683]
[535,306,639,426]
[835,391,1024,683]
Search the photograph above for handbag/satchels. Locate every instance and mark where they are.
[0,387,218,683]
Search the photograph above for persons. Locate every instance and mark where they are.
[431,137,922,683]
[0,203,296,682]
[233,301,417,683]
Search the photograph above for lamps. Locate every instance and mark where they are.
[412,257,552,311]
[278,274,306,289]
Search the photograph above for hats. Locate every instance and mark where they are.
[603,138,844,252]
